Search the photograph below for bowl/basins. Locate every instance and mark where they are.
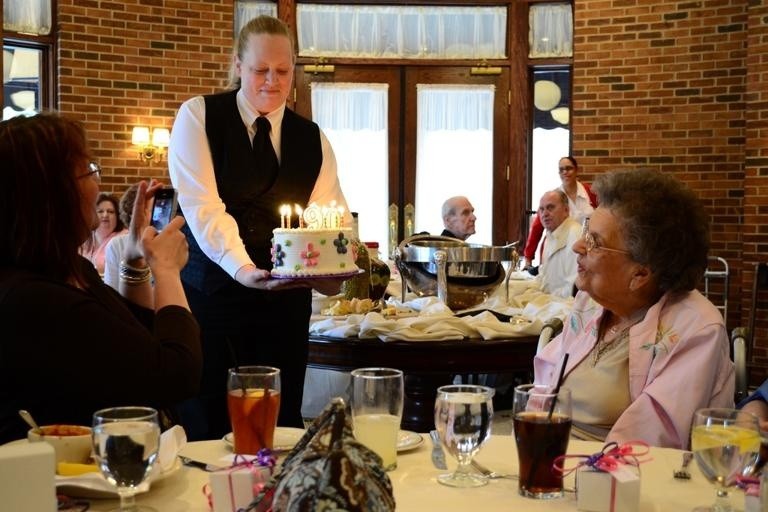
[27,425,92,474]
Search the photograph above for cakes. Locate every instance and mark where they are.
[272,229,356,275]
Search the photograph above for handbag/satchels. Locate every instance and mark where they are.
[241,398,396,512]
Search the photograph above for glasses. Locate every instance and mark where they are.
[74,162,102,186]
[583,218,633,256]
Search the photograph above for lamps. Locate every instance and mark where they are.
[154,126,171,148]
[132,127,151,148]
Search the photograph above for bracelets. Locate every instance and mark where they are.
[117,261,152,285]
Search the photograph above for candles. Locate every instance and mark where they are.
[296,202,304,231]
[286,203,291,231]
[340,206,347,229]
[322,208,329,229]
[279,204,284,228]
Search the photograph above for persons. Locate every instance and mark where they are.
[438,196,478,241]
[103,181,143,295]
[732,327,768,460]
[527,168,733,451]
[521,156,602,273]
[533,190,585,300]
[168,17,359,432]
[0,111,217,443]
[74,193,128,272]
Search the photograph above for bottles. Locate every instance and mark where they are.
[344,213,370,299]
[364,243,390,299]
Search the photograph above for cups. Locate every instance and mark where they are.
[691,408,761,511]
[513,384,573,500]
[227,365,281,456]
[433,385,496,489]
[91,406,160,511]
[350,367,405,473]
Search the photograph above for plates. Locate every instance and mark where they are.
[222,425,308,452]
[396,429,424,453]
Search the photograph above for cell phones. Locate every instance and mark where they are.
[150,189,178,234]
